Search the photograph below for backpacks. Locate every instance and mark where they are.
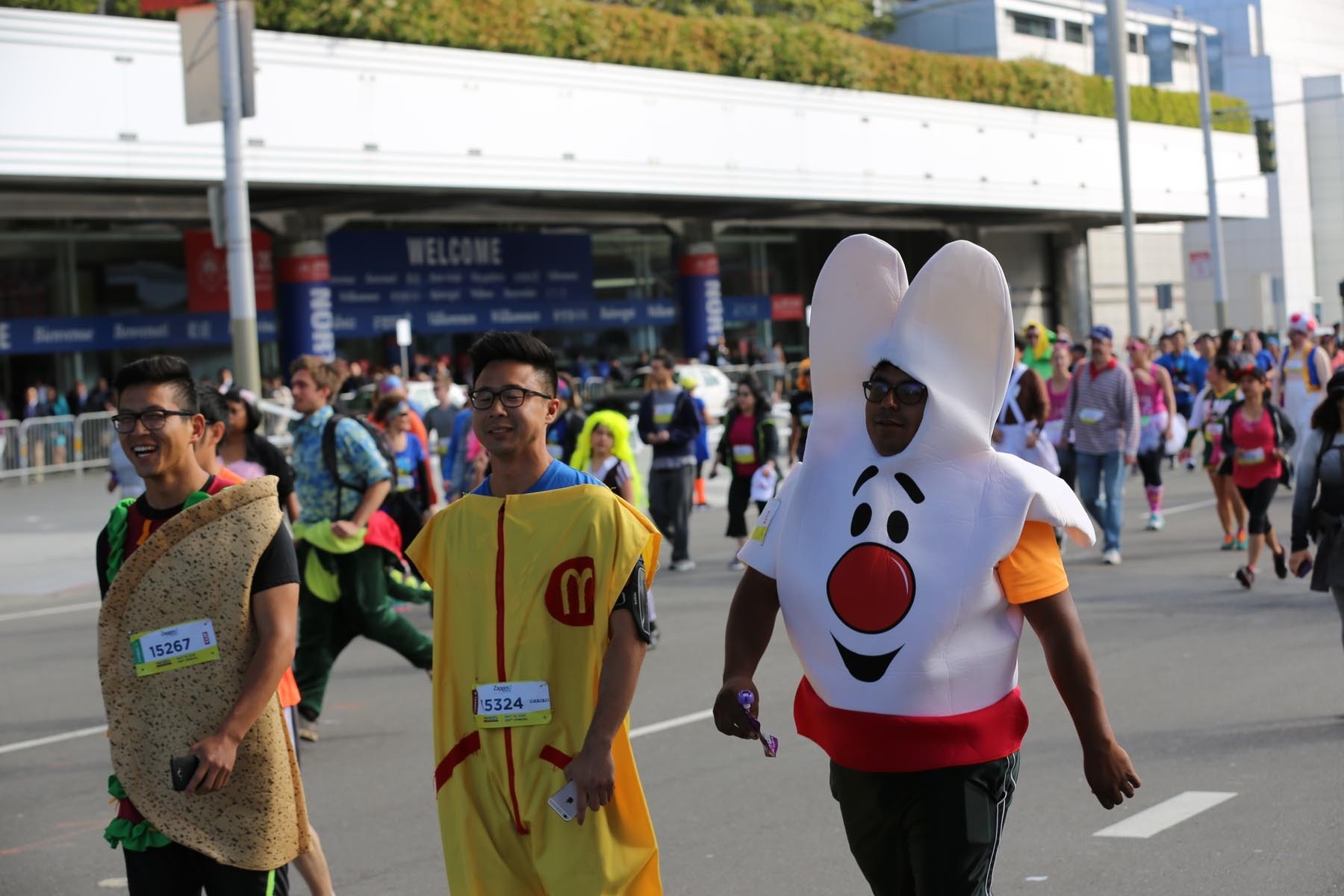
[323,407,398,522]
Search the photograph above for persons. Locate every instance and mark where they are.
[189,385,337,896]
[90,356,301,895]
[405,333,666,896]
[4,290,1343,628]
[265,350,440,740]
[712,333,1140,896]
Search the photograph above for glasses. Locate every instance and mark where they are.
[111,409,193,434]
[862,380,926,406]
[468,387,552,410]
